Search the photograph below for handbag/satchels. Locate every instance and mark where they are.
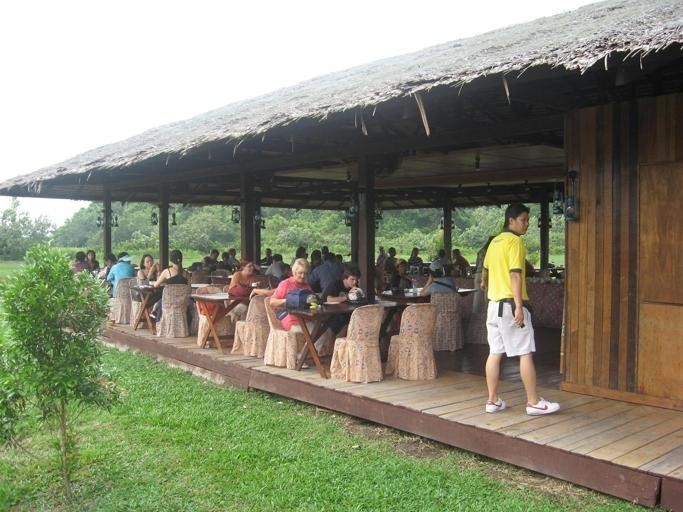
[229,288,247,296]
[286,288,323,310]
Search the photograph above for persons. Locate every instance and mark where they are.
[322,265,361,337]
[269,258,332,369]
[199,247,239,272]
[227,258,258,316]
[479,203,561,416]
[153,249,190,322]
[260,248,346,291]
[74,249,160,295]
[376,246,469,295]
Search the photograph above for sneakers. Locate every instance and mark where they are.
[486,397,505,413]
[526,397,560,415]
[296,359,308,368]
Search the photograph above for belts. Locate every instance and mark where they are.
[503,298,527,304]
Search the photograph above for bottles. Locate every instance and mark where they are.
[412,271,419,294]
[249,283,256,294]
[256,281,262,288]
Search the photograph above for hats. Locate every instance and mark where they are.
[430,263,440,271]
[119,254,133,262]
